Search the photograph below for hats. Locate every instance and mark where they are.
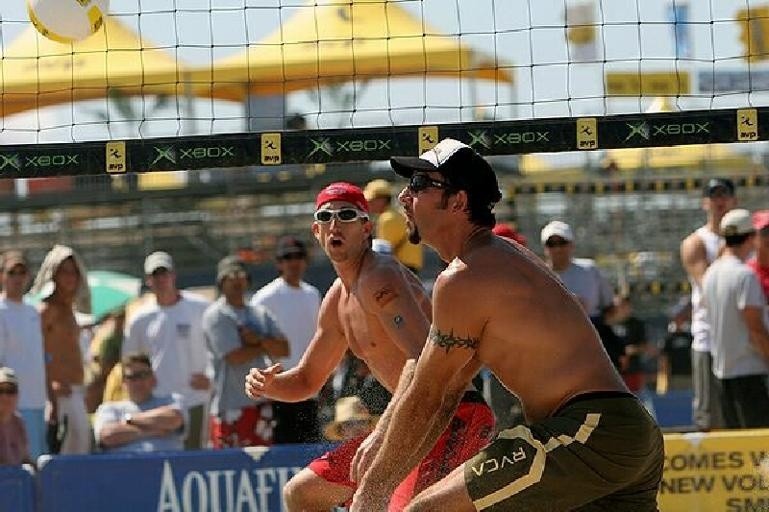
[702,178,769,236]
[0,251,29,272]
[0,367,19,385]
[324,396,380,441]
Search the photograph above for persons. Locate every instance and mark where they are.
[247,183,496,512]
[2,109,768,458]
[353,136,666,512]
